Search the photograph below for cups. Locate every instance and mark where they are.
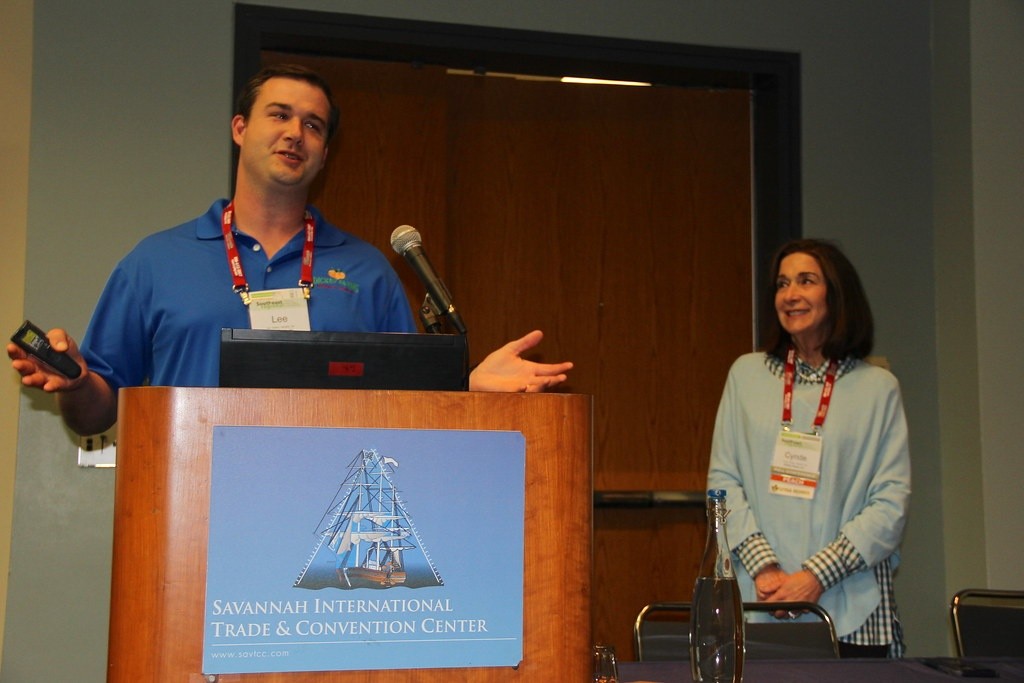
[593,643,618,683]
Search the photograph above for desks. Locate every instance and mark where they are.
[611,653,1024,683]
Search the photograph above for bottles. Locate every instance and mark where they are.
[690,489,745,682]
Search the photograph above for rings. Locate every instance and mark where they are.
[788,610,800,620]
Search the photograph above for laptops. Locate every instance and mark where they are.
[219,327,470,392]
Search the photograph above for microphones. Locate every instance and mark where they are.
[390,225,467,337]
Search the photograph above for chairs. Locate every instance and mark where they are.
[949,586,1023,656]
[627,596,841,659]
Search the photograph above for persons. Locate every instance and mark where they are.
[709,234,915,661]
[5,63,576,438]
